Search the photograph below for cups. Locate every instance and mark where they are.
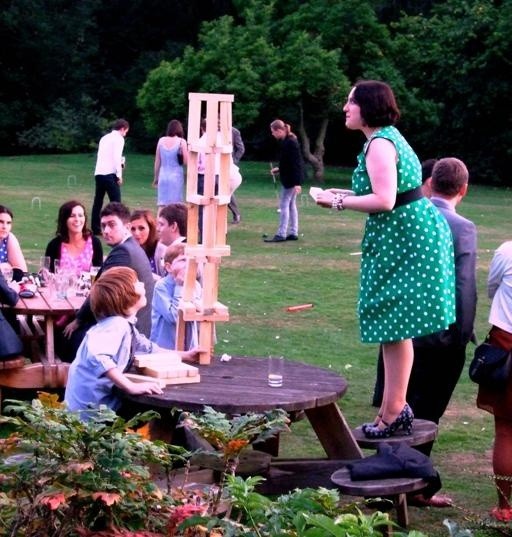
[40,256,50,272]
[268,356,284,387]
[54,277,68,300]
[121,156,125,168]
[54,259,61,274]
[90,266,102,284]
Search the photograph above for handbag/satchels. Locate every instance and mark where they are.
[469,327,512,389]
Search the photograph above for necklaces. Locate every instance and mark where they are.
[331,192,348,211]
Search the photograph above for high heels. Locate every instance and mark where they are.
[364,403,414,440]
[362,413,382,434]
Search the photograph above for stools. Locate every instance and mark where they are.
[330,468,431,537]
[353,419,439,455]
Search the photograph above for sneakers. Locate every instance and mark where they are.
[407,494,453,508]
[488,506,512,523]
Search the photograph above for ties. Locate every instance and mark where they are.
[122,322,137,374]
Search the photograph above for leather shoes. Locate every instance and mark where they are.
[264,235,286,243]
[233,215,240,224]
[285,234,298,241]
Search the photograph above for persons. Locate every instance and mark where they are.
[370,158,440,408]
[91,118,129,236]
[44,201,103,274]
[153,119,187,231]
[156,204,187,245]
[0,204,28,274]
[63,267,214,428]
[0,269,23,360]
[130,209,158,258]
[197,118,220,245]
[54,202,155,365]
[149,243,198,352]
[391,158,476,507]
[316,80,456,440]
[218,113,245,223]
[263,120,304,243]
[475,239,512,521]
